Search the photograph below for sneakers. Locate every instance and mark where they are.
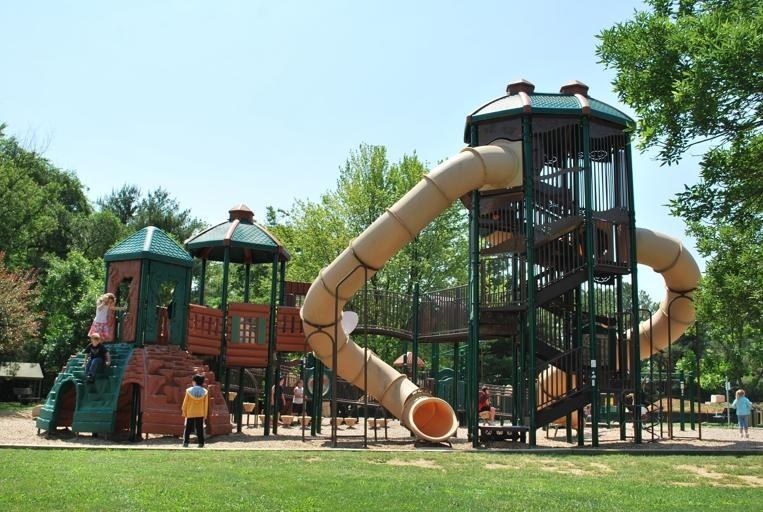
[741,433,748,438]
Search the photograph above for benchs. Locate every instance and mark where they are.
[477,425,533,444]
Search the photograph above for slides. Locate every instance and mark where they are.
[299,144,701,443]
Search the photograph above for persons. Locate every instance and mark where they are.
[88,293,127,344]
[731,389,753,438]
[181,373,208,447]
[271,376,287,426]
[82,333,111,378]
[479,386,495,421]
[291,380,307,416]
